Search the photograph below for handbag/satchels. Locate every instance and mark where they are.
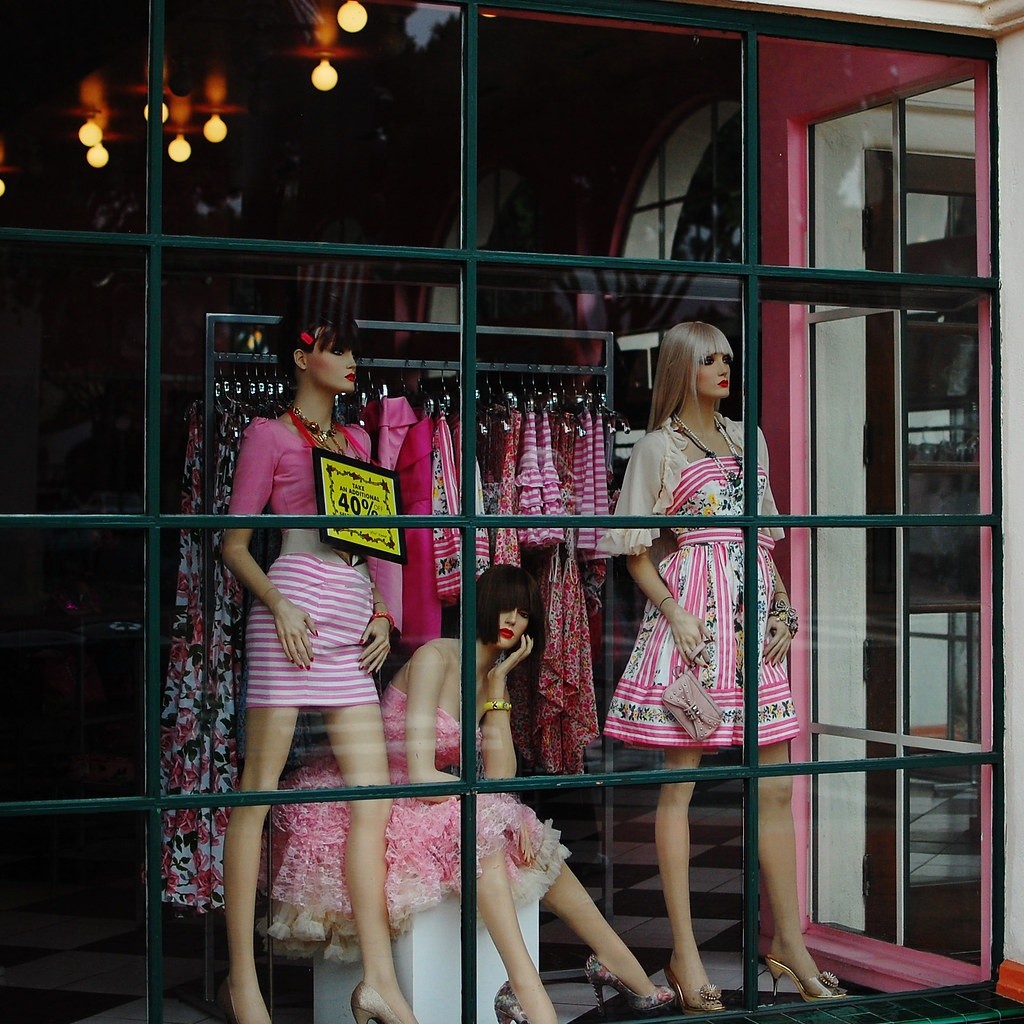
[662,644,723,743]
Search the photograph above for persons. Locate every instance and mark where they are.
[221,301,418,1024]
[596,322,847,1015]
[258,563,676,1024]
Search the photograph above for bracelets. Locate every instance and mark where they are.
[371,612,394,632]
[480,700,513,711]
[769,600,799,638]
[658,596,674,612]
[374,602,385,605]
[774,591,787,596]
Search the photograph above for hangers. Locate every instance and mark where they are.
[214,352,630,435]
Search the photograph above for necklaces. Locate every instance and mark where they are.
[672,414,744,502]
[289,403,337,442]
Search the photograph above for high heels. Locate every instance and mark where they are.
[765,954,847,1003]
[495,980,531,1024]
[664,964,726,1014]
[584,953,678,1015]
[350,981,402,1024]
[225,973,272,1024]
